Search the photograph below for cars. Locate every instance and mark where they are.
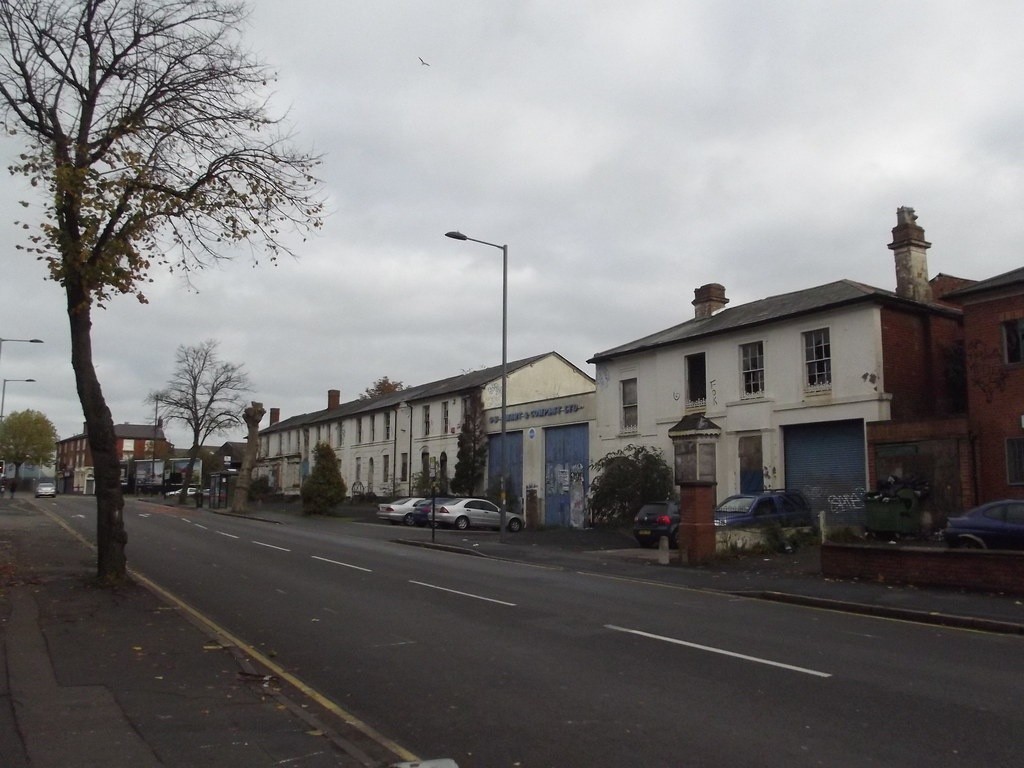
[35,482,56,498]
[165,488,225,496]
[378,498,452,528]
[428,498,525,532]
[944,500,1024,550]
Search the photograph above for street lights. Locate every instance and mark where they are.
[445,232,508,543]
[0,379,36,421]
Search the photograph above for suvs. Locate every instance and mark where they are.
[714,489,811,531]
[632,501,681,550]
